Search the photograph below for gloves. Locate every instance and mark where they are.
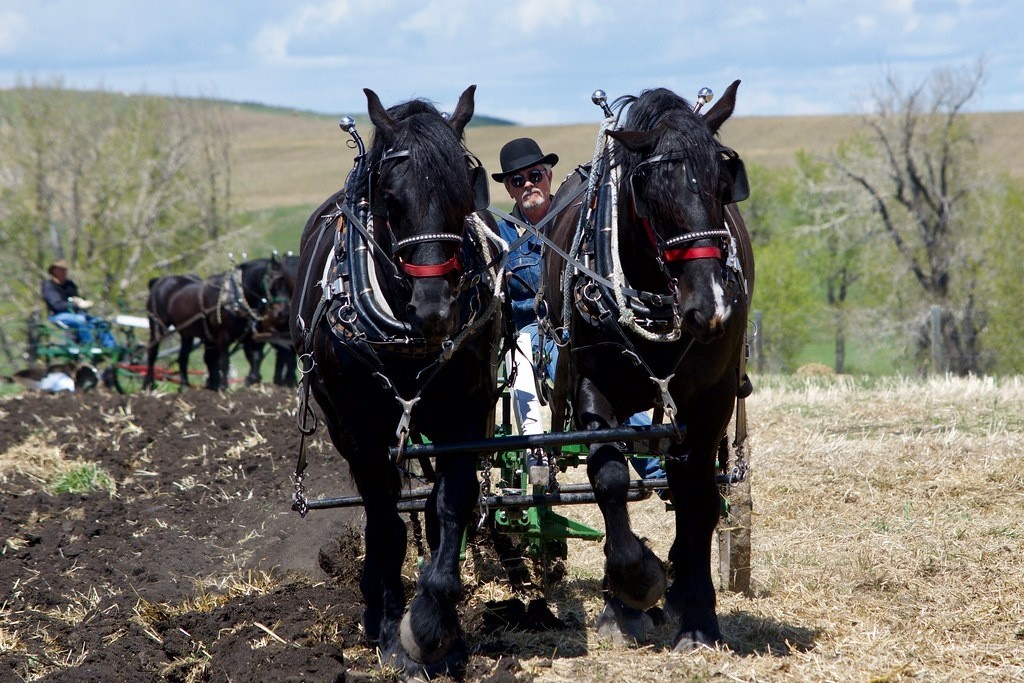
[72,296,94,310]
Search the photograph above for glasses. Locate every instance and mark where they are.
[507,169,546,189]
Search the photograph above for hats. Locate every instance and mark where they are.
[47,259,71,275]
[491,138,559,183]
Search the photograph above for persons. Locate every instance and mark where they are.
[41,258,120,354]
[491,137,670,501]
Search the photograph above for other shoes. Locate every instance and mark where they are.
[77,356,93,364]
[654,476,669,501]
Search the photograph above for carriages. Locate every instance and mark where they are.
[293,76,753,675]
[7,254,300,393]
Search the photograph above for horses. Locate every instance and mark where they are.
[541,77,757,655]
[141,252,300,394]
[295,86,507,674]
